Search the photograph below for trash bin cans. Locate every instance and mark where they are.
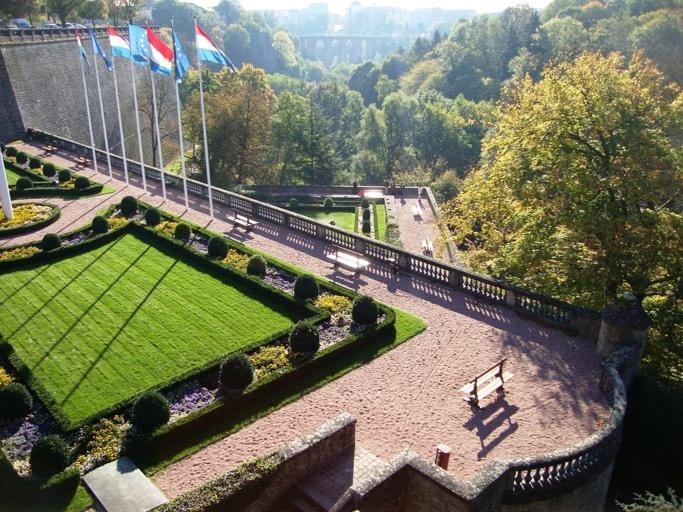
[435,443,451,470]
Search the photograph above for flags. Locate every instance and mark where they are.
[106,25,130,62]
[74,30,92,69]
[192,22,237,76]
[144,23,173,81]
[86,25,112,74]
[170,29,190,85]
[128,23,148,67]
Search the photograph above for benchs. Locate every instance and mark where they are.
[326,244,369,277]
[42,141,58,154]
[225,207,259,232]
[459,367,515,410]
[72,152,91,168]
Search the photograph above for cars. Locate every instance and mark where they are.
[3,18,89,28]
